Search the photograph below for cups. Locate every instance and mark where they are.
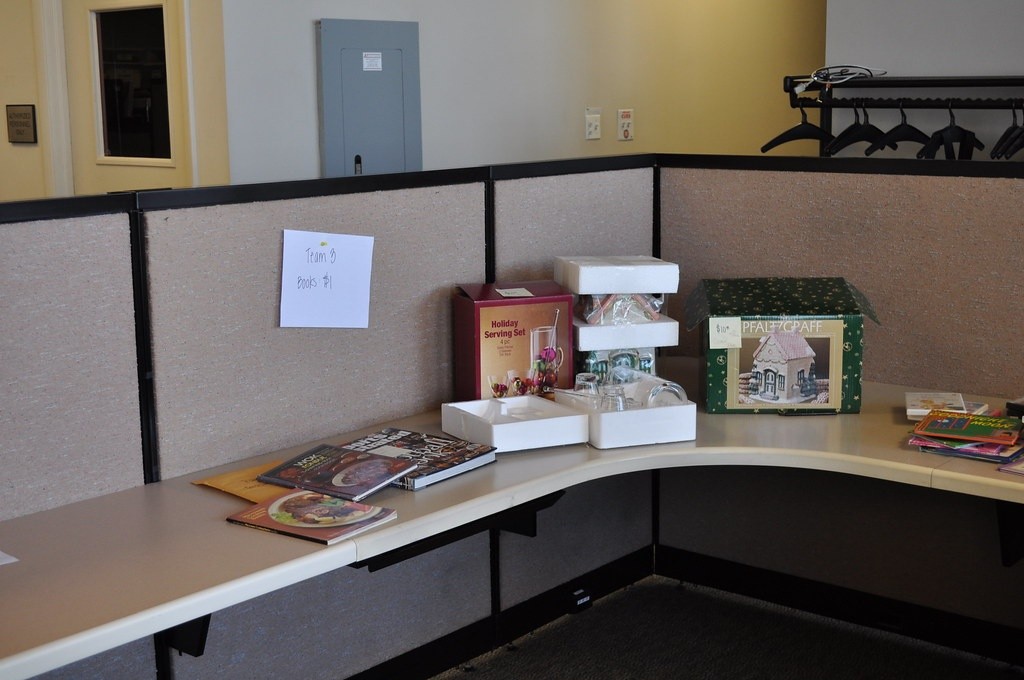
[488,375,510,398]
[574,373,599,395]
[507,368,536,396]
[602,365,688,409]
[529,325,564,392]
[600,385,629,411]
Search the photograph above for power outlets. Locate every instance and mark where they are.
[586,107,602,140]
[617,109,635,141]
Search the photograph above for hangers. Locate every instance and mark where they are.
[916,100,985,159]
[760,96,835,154]
[865,100,940,156]
[989,100,1024,159]
[823,97,898,156]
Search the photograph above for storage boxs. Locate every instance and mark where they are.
[441,394,590,453]
[573,312,679,352]
[581,348,657,386]
[450,279,575,402]
[554,381,698,450]
[684,277,882,415]
[553,255,680,295]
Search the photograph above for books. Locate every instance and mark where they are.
[903,391,1024,477]
[226,488,398,545]
[338,429,497,491]
[256,443,418,501]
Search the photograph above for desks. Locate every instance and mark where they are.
[0,455,355,680]
[263,380,1011,573]
[932,401,1024,567]
